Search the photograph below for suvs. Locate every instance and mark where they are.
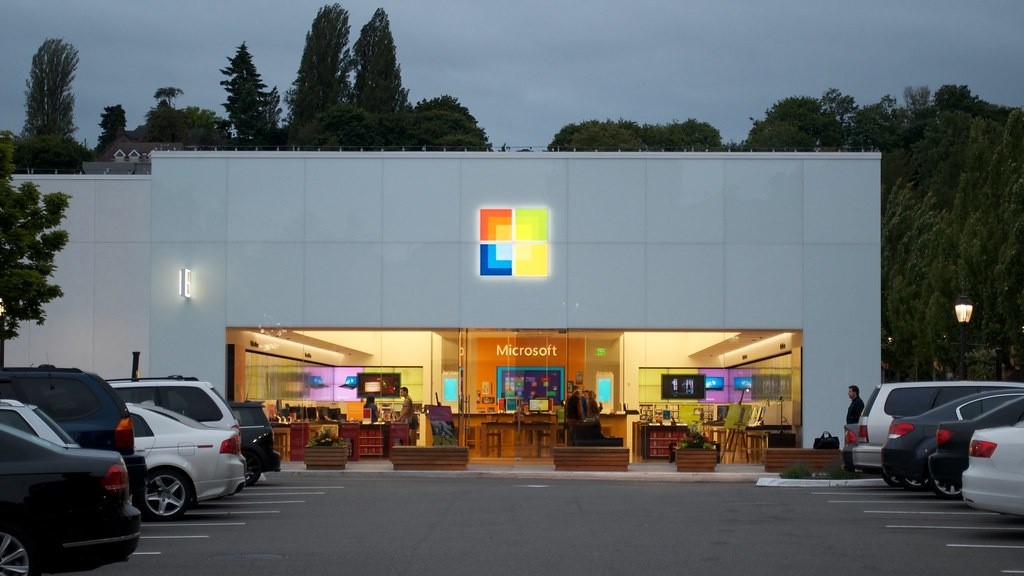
[0,399,82,449]
[852,380,1024,489]
[0,363,151,514]
[104,374,249,522]
[226,400,282,488]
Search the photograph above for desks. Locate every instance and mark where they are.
[481,422,557,458]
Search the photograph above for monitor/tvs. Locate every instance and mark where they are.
[734,377,752,390]
[706,377,724,390]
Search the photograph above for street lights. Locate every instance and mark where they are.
[954,294,975,380]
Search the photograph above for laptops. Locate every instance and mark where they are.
[309,377,330,389]
[338,376,357,390]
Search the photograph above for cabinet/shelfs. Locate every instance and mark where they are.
[643,424,690,461]
[360,423,409,458]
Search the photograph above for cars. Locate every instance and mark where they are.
[961,419,1024,517]
[929,395,1024,500]
[841,423,858,472]
[0,423,143,576]
[879,387,1024,492]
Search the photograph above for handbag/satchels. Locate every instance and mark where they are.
[813,431,840,449]
[410,416,419,430]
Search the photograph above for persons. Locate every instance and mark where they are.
[567,386,603,446]
[844,385,864,472]
[364,397,382,422]
[397,386,416,446]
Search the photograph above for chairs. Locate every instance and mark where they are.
[465,425,479,457]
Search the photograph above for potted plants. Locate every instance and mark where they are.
[303,433,350,470]
[673,432,717,472]
